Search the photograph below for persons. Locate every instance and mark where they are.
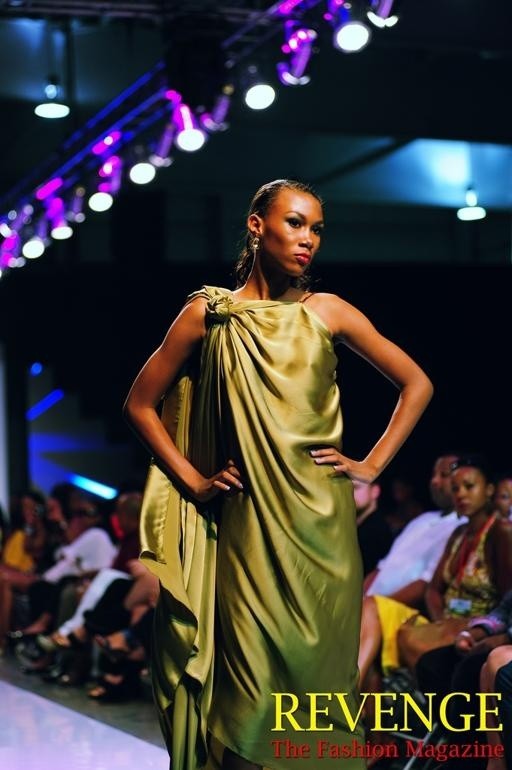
[122,178,436,770]
[1,448,512,769]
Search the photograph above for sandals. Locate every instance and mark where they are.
[6,631,131,699]
